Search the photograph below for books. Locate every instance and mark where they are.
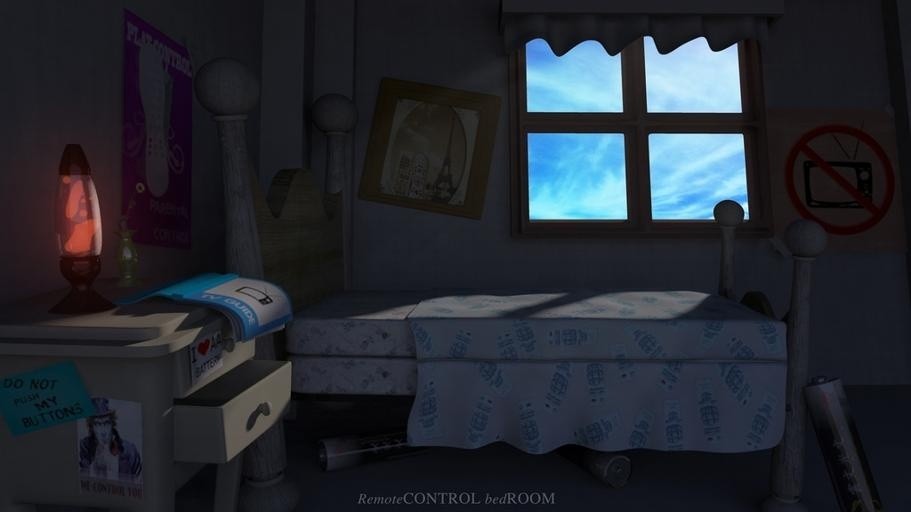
[111,270,294,341]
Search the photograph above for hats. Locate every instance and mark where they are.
[89,398,111,417]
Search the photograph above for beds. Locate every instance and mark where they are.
[195,58,827,512]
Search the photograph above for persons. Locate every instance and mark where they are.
[77,396,143,496]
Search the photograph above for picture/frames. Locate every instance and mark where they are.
[357,77,505,221]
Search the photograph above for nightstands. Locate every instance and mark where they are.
[0,276,293,512]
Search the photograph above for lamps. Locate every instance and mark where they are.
[48,142,119,317]
